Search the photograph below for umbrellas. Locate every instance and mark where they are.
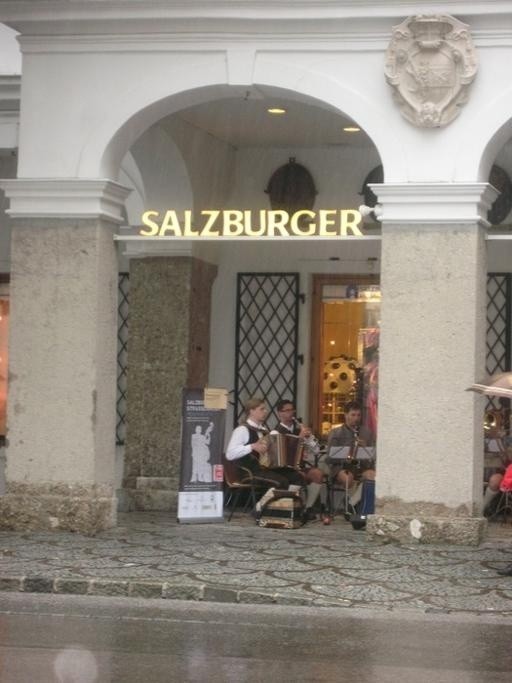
[463,371,511,399]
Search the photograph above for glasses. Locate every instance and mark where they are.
[280,408,297,413]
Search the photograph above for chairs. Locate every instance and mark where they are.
[222,454,263,524]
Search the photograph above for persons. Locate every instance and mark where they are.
[271,398,325,520]
[484,452,511,513]
[326,401,375,520]
[226,397,305,525]
